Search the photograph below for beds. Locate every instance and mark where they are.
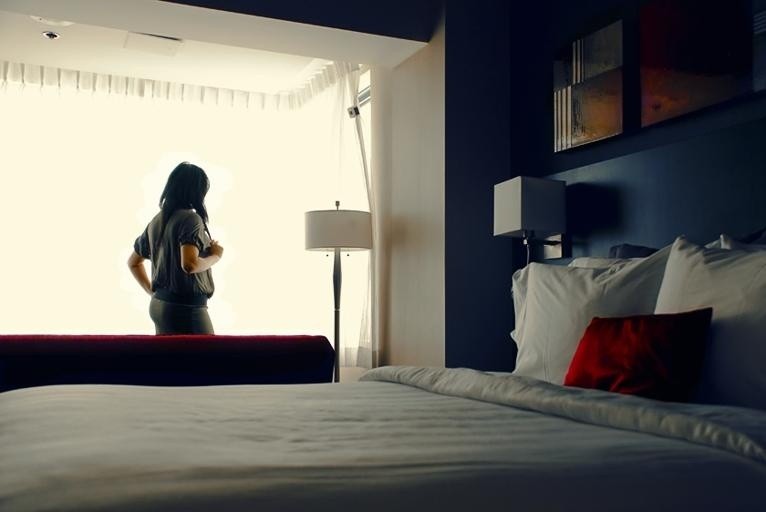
[0,117,766,512]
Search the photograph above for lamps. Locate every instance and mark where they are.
[306,201,373,382]
[493,175,567,264]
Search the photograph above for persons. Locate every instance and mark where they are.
[127,160,223,335]
